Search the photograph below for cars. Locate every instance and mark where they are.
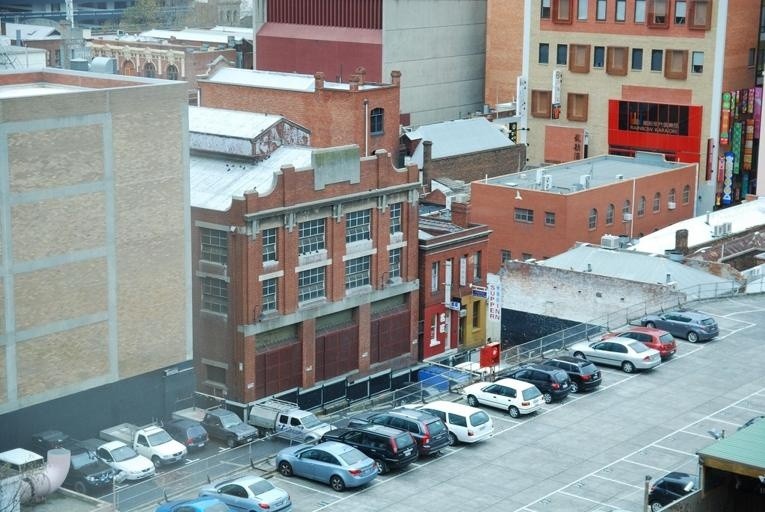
[638,312,720,343]
[646,471,702,511]
[275,440,378,491]
[565,339,660,373]
[462,378,545,417]
[197,472,291,512]
[150,496,233,511]
[605,327,676,358]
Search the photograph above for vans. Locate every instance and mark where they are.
[395,400,493,446]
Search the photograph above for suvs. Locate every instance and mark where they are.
[546,356,601,392]
[348,406,449,458]
[503,366,572,404]
[321,422,417,475]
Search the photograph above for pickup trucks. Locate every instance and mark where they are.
[32,398,329,494]
